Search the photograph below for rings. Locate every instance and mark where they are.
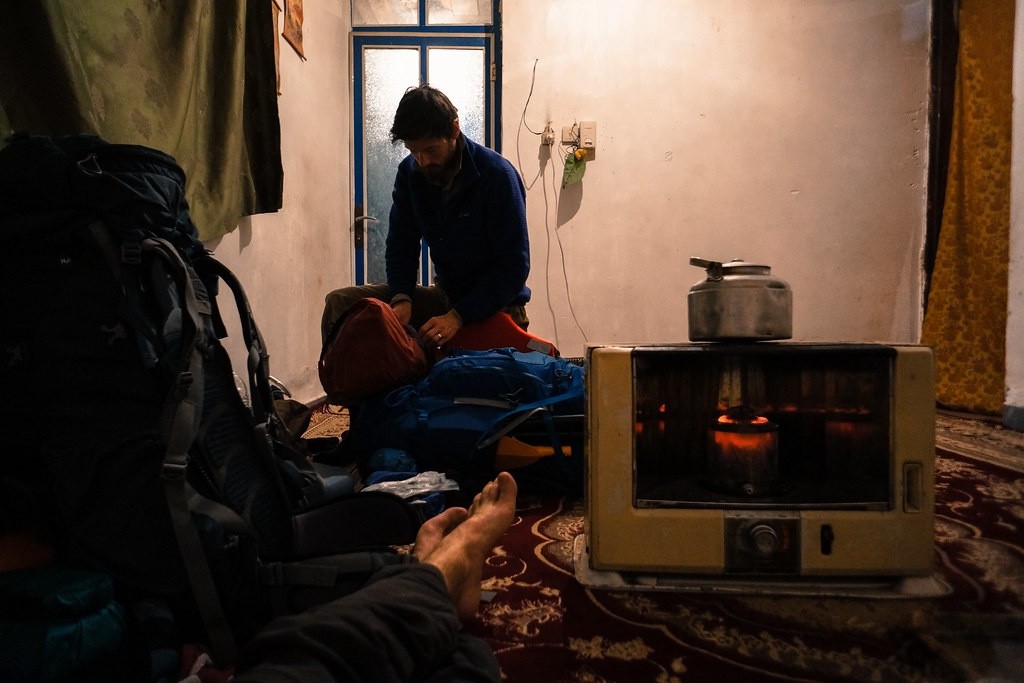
[438,334,442,339]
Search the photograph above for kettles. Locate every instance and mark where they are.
[685,255,795,340]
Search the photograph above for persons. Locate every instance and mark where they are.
[320,84,533,390]
[233,470,518,683]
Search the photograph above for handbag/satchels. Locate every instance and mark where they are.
[319,298,431,400]
[346,346,586,477]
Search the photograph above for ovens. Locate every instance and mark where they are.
[588,347,936,575]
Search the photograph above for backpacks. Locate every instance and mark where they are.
[0,119,327,657]
